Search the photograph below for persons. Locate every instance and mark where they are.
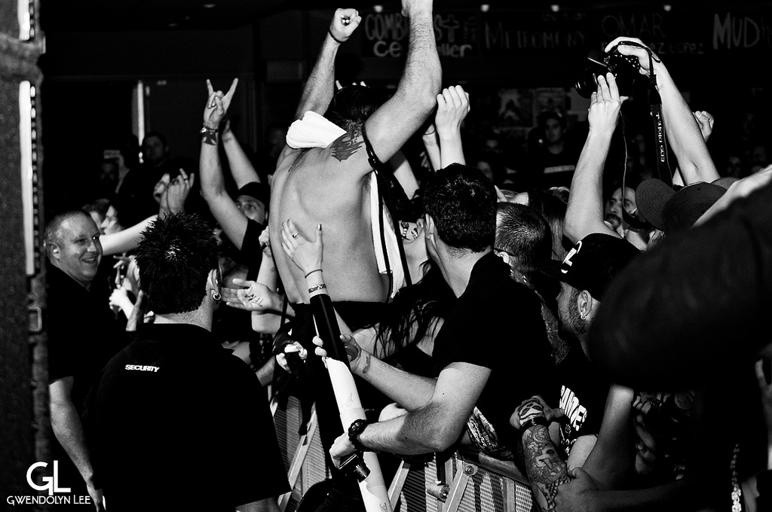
[42,0,772,512]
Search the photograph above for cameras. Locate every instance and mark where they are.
[575,46,662,107]
[338,453,371,482]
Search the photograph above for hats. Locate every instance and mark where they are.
[634,178,728,232]
[542,232,640,303]
[235,179,269,209]
